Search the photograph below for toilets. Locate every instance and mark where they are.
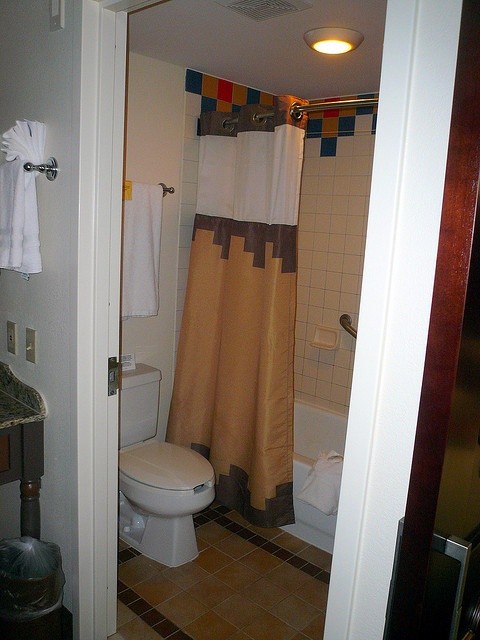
[119,440,216,567]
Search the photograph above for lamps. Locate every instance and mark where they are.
[303,26,365,57]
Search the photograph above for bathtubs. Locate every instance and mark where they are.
[278,398,347,555]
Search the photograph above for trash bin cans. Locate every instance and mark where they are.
[0,536,66,640]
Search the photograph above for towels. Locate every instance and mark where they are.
[123,181,163,320]
[0,159,42,281]
[297,450,344,518]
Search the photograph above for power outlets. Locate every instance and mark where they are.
[5,321,20,354]
